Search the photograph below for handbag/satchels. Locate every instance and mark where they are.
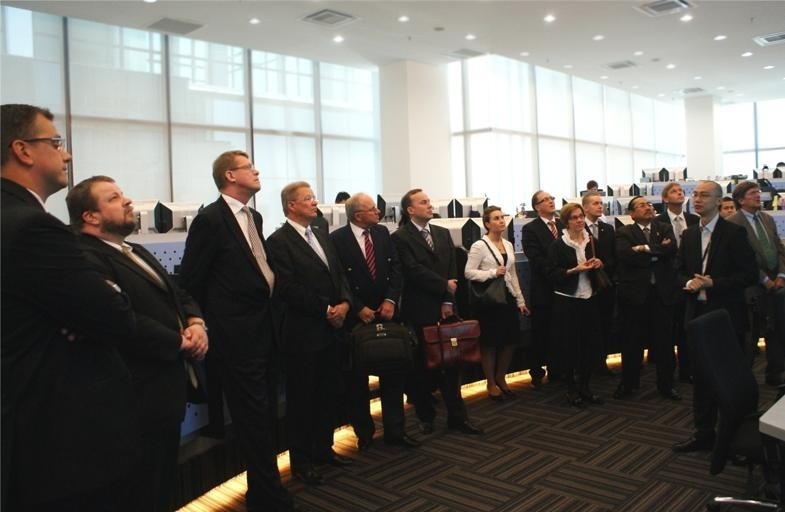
[421,313,483,371]
[467,238,509,313]
[751,286,785,338]
[588,235,613,294]
[345,312,418,378]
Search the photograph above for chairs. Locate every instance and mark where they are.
[761,286,784,404]
[679,305,785,511]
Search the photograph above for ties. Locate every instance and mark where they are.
[121,245,198,389]
[643,227,651,245]
[305,228,331,272]
[752,215,778,272]
[362,229,377,281]
[242,206,275,299]
[547,221,559,241]
[675,216,684,249]
[421,227,435,252]
[591,224,599,240]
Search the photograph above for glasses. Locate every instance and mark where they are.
[743,190,766,192]
[539,196,560,203]
[8,136,66,152]
[692,193,718,197]
[233,166,261,172]
[290,196,315,204]
[359,207,379,212]
[636,204,655,208]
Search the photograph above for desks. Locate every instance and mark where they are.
[755,391,784,447]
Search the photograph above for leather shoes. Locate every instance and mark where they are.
[446,418,484,436]
[292,465,326,487]
[766,369,785,390]
[671,432,717,452]
[417,421,433,435]
[314,446,354,466]
[731,452,754,466]
[245,488,300,512]
[531,372,708,406]
[487,384,516,401]
[359,432,422,448]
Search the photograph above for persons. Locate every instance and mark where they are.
[181,150,300,511]
[62,169,214,512]
[0,99,139,512]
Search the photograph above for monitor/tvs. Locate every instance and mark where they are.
[563,164,785,244]
[121,196,204,279]
[428,194,539,254]
[316,193,403,236]
